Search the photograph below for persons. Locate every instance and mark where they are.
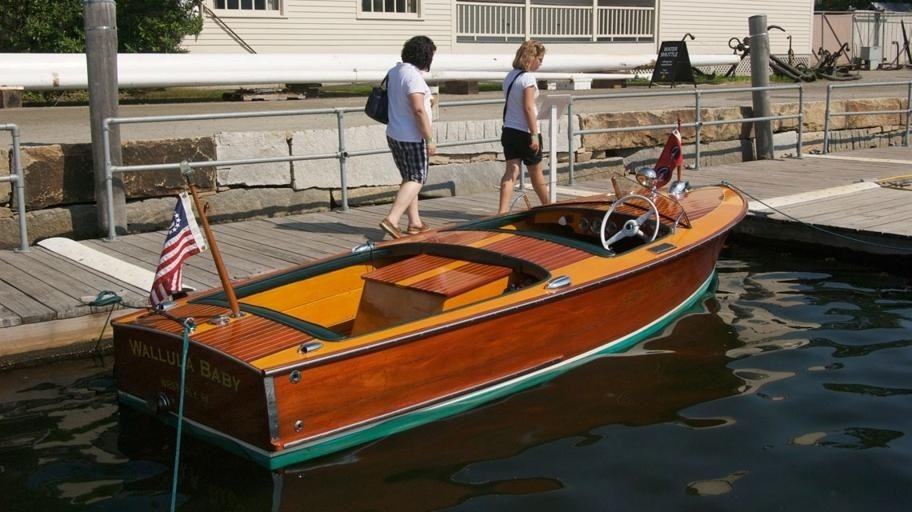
[378,36,437,243]
[498,41,551,215]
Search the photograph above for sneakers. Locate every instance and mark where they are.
[379,219,401,239]
[408,222,431,234]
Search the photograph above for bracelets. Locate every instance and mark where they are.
[425,138,433,144]
[531,135,539,140]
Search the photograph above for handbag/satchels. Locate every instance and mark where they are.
[364,75,388,123]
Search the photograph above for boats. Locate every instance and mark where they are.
[90,296,732,510]
[106,120,750,477]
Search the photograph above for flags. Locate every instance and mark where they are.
[654,130,682,191]
[148,192,208,310]
